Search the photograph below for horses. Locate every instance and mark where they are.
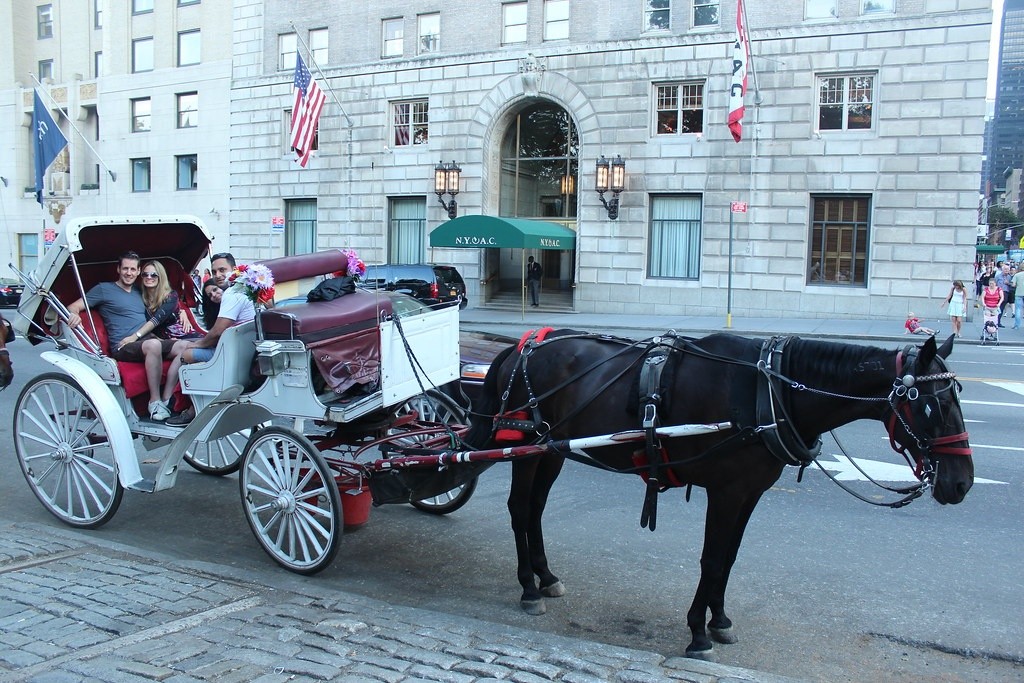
[469,327,975,664]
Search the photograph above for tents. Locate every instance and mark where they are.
[430,214,577,320]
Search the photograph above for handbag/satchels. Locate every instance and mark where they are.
[948,287,955,303]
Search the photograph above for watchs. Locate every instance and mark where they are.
[134,331,142,338]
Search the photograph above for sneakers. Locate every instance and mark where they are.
[165,409,194,426]
[148,400,172,421]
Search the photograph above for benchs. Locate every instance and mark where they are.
[180,319,260,396]
[61,300,210,399]
[251,250,392,348]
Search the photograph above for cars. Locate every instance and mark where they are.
[0,278,25,306]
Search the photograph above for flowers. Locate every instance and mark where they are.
[329,249,366,288]
[226,263,274,310]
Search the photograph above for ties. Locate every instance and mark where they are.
[530,264,533,271]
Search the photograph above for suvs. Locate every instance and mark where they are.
[355,263,468,311]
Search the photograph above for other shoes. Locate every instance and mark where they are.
[935,330,940,334]
[533,304,539,308]
[1011,312,1015,318]
[1003,312,1008,316]
[928,333,934,336]
[1011,326,1018,329]
[954,334,957,337]
[999,325,1005,328]
[530,303,535,306]
[958,335,962,337]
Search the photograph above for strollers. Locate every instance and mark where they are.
[981,305,1000,346]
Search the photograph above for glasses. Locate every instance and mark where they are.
[218,253,227,258]
[140,271,159,278]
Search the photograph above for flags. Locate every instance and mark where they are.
[33,87,69,209]
[290,48,328,168]
[728,0,749,142]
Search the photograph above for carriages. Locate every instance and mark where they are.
[10,214,974,662]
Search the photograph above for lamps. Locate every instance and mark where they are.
[594,153,625,220]
[433,160,462,220]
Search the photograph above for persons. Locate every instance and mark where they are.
[527,256,542,308]
[981,278,1004,315]
[114,258,206,352]
[974,259,1024,330]
[164,252,267,427]
[194,270,201,290]
[202,268,211,284]
[202,277,229,332]
[903,312,940,336]
[940,280,967,338]
[66,249,194,420]
